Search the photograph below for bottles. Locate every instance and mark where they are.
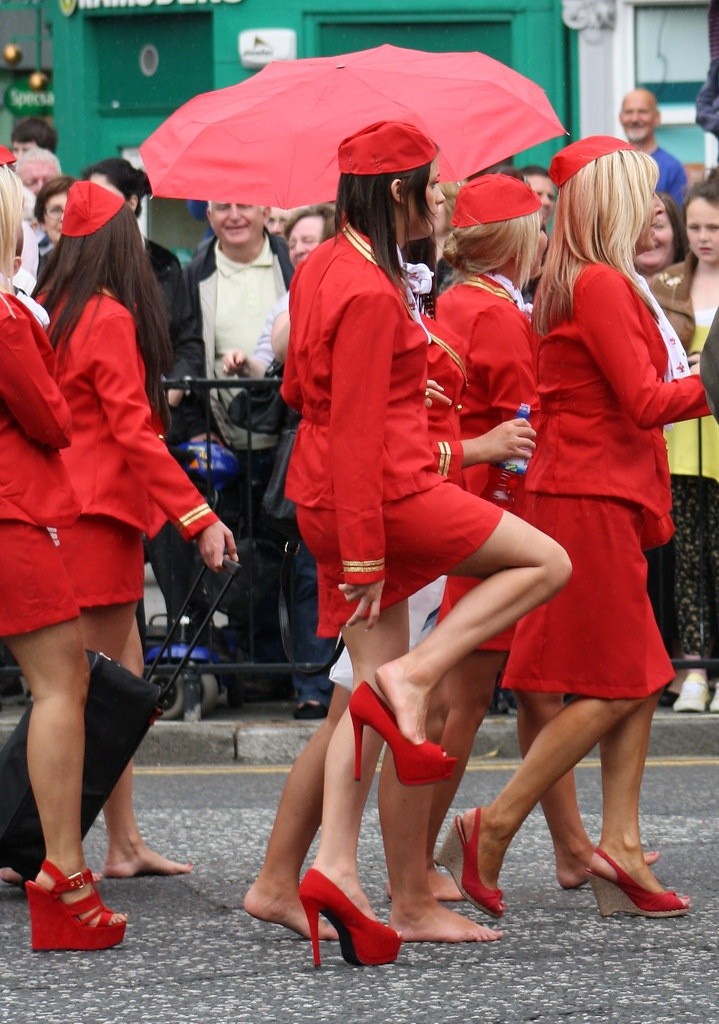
[479,402,531,513]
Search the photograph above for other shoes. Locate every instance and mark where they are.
[294,702,327,720]
[673,671,719,713]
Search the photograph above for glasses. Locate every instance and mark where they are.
[44,206,65,219]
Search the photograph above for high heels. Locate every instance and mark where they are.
[584,847,690,919]
[25,860,127,951]
[299,868,404,970]
[433,806,506,918]
[347,680,459,786]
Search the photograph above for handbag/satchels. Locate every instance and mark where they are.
[262,412,300,521]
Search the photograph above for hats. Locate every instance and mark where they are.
[338,118,438,174]
[450,173,544,226]
[547,136,637,187]
[59,180,124,237]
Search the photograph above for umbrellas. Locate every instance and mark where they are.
[137,42,570,217]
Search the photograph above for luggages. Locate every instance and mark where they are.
[0,560,242,884]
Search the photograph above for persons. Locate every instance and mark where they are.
[695,56,719,139]
[620,88,689,207]
[1,117,719,972]
